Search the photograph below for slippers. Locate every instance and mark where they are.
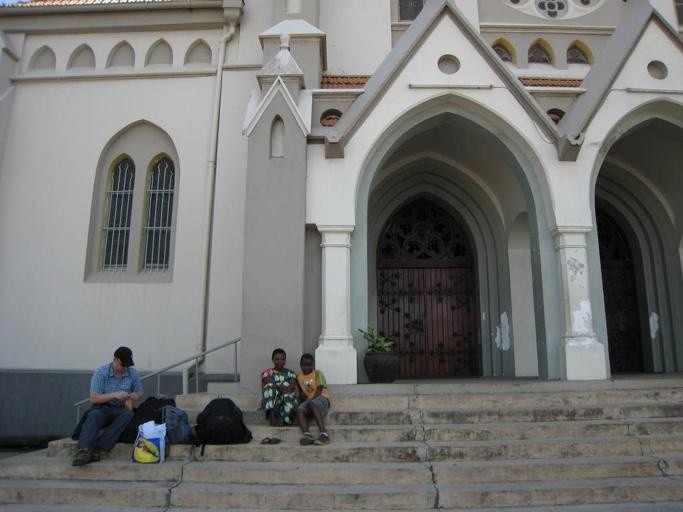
[260,437,280,444]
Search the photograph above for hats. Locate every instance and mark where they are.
[114,347,134,367]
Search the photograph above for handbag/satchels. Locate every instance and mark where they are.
[195,398,253,444]
[118,396,193,464]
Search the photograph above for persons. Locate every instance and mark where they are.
[72,346,143,466]
[256,349,302,426]
[297,354,330,445]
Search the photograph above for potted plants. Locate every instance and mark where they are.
[357,325,401,383]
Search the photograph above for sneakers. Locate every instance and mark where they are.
[72,448,101,466]
[314,434,329,445]
[300,434,313,445]
